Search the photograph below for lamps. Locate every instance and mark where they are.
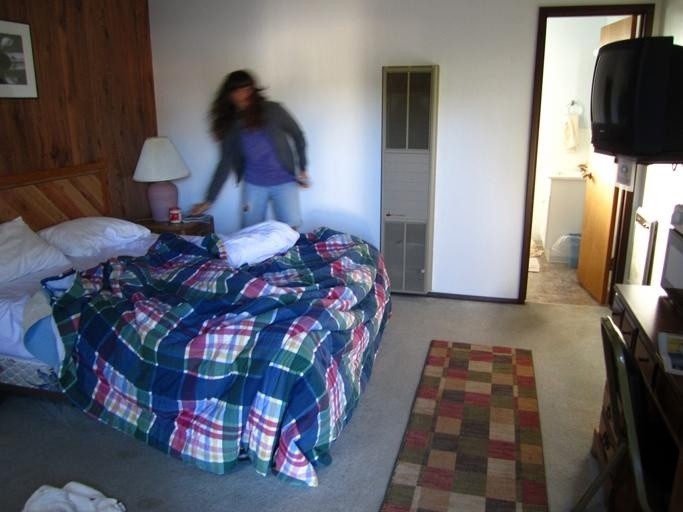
[132,135,191,222]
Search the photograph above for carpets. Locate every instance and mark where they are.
[380,340,550,512]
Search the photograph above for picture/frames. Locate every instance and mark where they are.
[0,20,40,99]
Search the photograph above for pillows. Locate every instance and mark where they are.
[1,214,154,283]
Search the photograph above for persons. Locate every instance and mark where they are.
[185,70,309,234]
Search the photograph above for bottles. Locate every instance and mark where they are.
[169,206,183,224]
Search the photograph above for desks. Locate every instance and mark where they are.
[590,283,683,512]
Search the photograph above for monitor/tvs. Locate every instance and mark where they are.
[590,36,683,155]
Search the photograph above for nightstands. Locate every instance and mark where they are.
[134,214,215,238]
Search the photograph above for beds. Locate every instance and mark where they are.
[1,161,391,491]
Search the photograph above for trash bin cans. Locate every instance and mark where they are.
[570,234,581,269]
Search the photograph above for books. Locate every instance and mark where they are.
[184,212,208,220]
[181,217,210,225]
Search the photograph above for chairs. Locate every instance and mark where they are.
[571,315,670,512]
